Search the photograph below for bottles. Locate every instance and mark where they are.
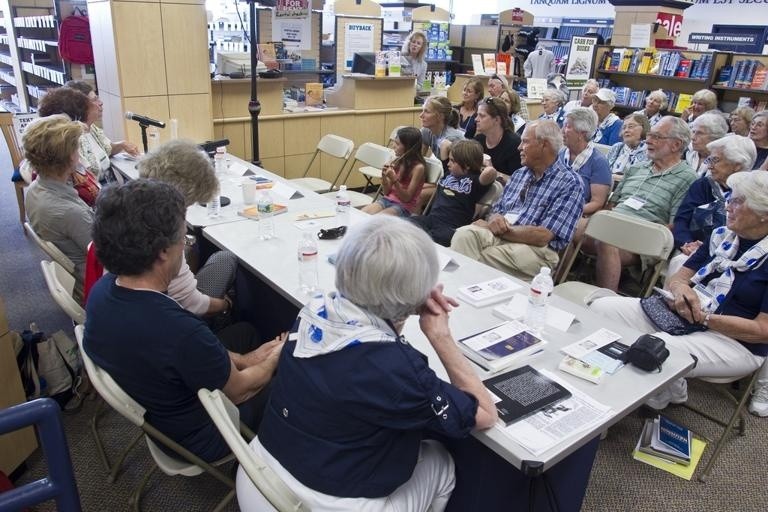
[257,190,275,240]
[297,231,320,294]
[627,49,642,74]
[214,147,227,179]
[335,185,350,228]
[522,267,553,334]
[148,124,159,154]
[206,195,221,218]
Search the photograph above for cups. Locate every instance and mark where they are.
[375,51,388,77]
[241,180,257,205]
[423,80,431,90]
[484,154,492,168]
[388,50,400,77]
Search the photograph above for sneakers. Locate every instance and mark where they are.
[646,377,688,409]
[748,379,768,417]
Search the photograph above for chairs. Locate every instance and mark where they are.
[639,349,768,482]
[0,112,28,224]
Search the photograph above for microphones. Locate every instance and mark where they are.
[125,111,166,169]
[197,140,230,207]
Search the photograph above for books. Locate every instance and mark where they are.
[237,203,288,221]
[493,366,571,428]
[249,176,272,189]
[599,46,768,120]
[238,203,287,221]
[632,415,707,482]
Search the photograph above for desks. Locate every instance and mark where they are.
[201,193,698,512]
[109,140,319,231]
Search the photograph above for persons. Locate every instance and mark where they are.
[83,138,238,332]
[22,80,140,266]
[82,180,288,463]
[234,216,498,512]
[361,74,768,410]
[399,29,428,91]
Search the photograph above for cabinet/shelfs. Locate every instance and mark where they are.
[591,45,768,110]
[325,0,383,92]
[0,0,71,118]
[208,0,324,91]
[384,2,454,73]
[451,9,535,85]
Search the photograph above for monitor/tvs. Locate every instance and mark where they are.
[217,52,268,78]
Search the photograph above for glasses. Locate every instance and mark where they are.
[520,176,534,203]
[182,232,197,247]
[491,74,503,83]
[486,98,500,116]
[646,132,678,142]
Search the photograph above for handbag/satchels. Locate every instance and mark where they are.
[641,294,708,335]
[30,322,79,395]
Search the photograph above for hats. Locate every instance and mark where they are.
[591,89,617,103]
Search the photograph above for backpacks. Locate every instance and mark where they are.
[58,7,93,65]
